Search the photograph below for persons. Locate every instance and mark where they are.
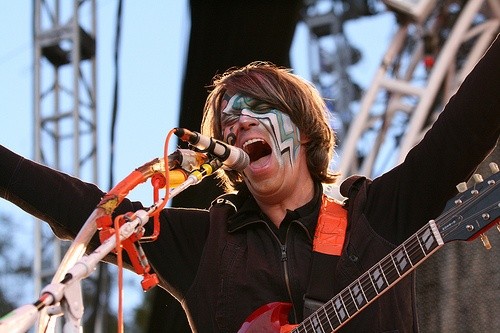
[0,31,500,333]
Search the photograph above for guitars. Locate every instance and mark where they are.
[236,162,500,333]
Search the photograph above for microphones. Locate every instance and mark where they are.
[151,127,250,188]
